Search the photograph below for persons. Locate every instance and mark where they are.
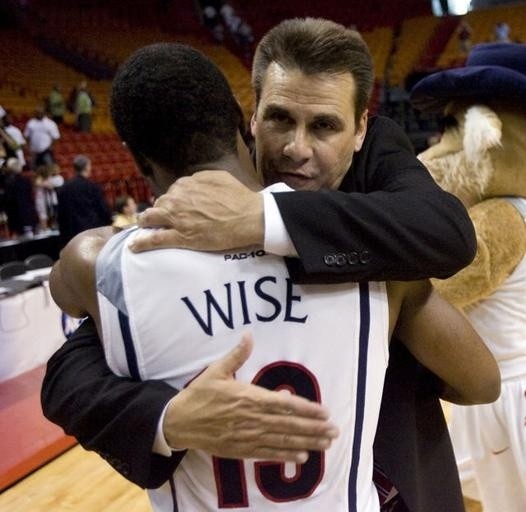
[0,74,141,241]
[494,22,510,43]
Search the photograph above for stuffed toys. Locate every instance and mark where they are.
[414,44,526,512]
